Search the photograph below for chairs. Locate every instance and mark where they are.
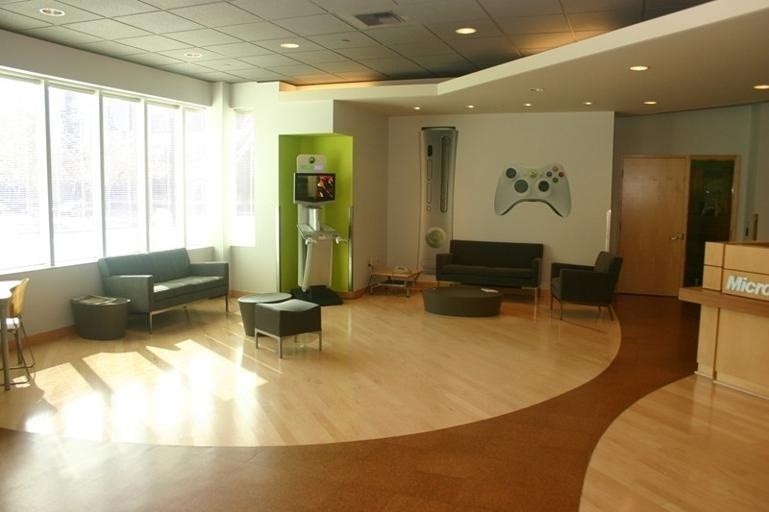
[551,251,623,320]
[0,278,36,384]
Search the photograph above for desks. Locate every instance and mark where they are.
[0,280,24,390]
[370,270,423,297]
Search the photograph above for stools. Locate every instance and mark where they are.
[238,293,292,336]
[72,296,132,339]
[254,299,322,359]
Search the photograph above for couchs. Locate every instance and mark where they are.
[435,240,543,303]
[98,248,229,334]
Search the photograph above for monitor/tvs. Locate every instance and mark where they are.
[293,172,336,209]
